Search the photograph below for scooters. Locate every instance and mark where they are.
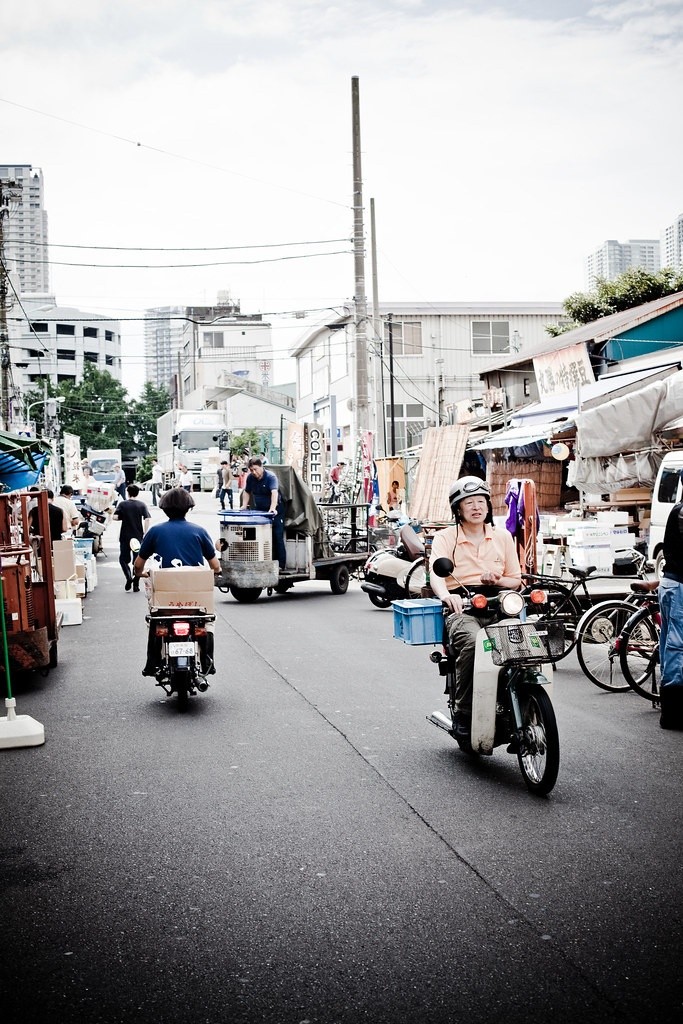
[331,499,404,549]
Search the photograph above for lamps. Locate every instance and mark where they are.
[551,441,569,461]
[9,363,31,369]
[7,345,44,357]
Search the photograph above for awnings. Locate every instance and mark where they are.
[396,419,577,454]
[0,429,52,475]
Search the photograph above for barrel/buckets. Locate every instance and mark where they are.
[74,538,93,559]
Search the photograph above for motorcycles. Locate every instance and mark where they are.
[78,500,109,560]
[359,547,425,609]
[128,537,230,714]
[424,556,565,800]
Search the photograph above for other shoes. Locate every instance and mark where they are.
[454,715,471,736]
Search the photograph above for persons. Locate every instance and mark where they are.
[112,485,152,592]
[216,450,270,512]
[81,458,96,482]
[430,476,522,737]
[328,462,347,503]
[134,488,222,677]
[9,485,79,557]
[240,458,286,570]
[112,464,126,508]
[658,468,683,732]
[178,465,194,511]
[387,480,402,512]
[152,458,164,506]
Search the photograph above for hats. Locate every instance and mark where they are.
[112,464,120,468]
[157,488,195,507]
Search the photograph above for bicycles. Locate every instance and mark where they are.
[517,549,663,710]
[344,526,401,577]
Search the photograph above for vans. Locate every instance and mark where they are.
[646,451,683,577]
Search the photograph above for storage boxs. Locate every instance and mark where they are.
[492,510,651,580]
[151,566,214,592]
[153,590,214,614]
[35,481,115,627]
[391,598,444,646]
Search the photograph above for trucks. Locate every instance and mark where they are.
[87,449,123,492]
[155,407,228,491]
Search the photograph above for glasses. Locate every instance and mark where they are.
[449,481,490,503]
[449,476,490,508]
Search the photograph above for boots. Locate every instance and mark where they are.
[659,685,683,730]
[199,631,216,673]
[132,568,140,592]
[142,633,162,676]
[123,567,133,591]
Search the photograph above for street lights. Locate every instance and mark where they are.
[0,303,56,430]
[27,395,65,431]
